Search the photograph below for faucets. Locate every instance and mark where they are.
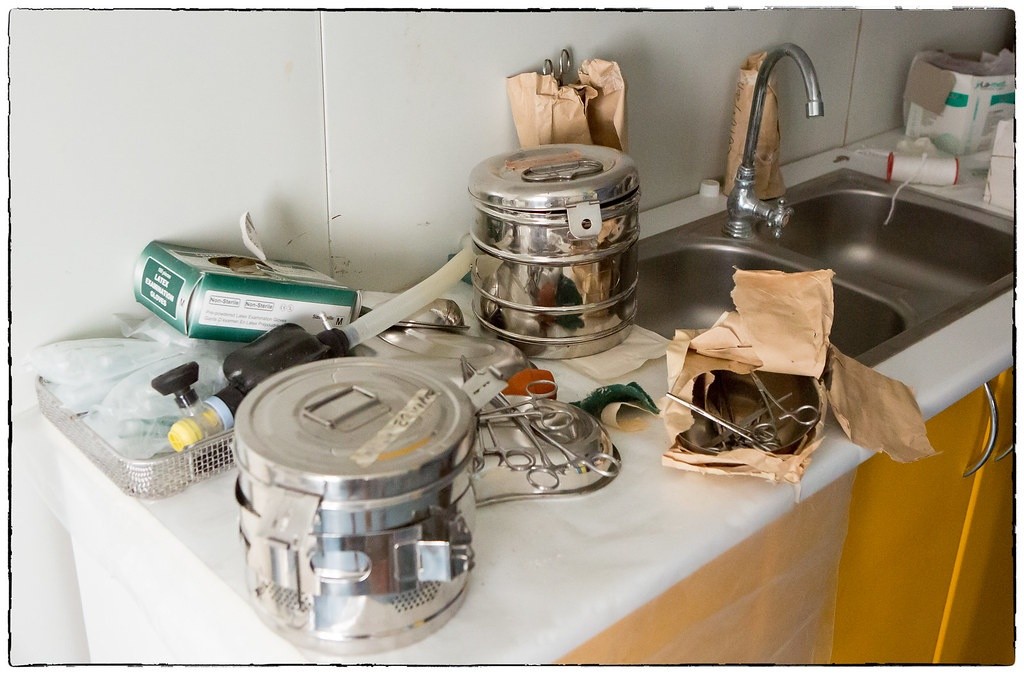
[721,43,824,239]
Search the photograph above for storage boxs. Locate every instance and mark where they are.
[902,45,1015,156]
[983,118,1015,212]
[133,208,358,342]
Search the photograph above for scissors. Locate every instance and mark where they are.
[664,368,820,452]
[457,355,622,491]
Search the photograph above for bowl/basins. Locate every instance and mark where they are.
[468,147,645,359]
[229,357,477,532]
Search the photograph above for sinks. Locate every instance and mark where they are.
[633,239,918,369]
[755,167,1015,294]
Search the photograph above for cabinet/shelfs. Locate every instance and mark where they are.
[831,366,1014,665]
